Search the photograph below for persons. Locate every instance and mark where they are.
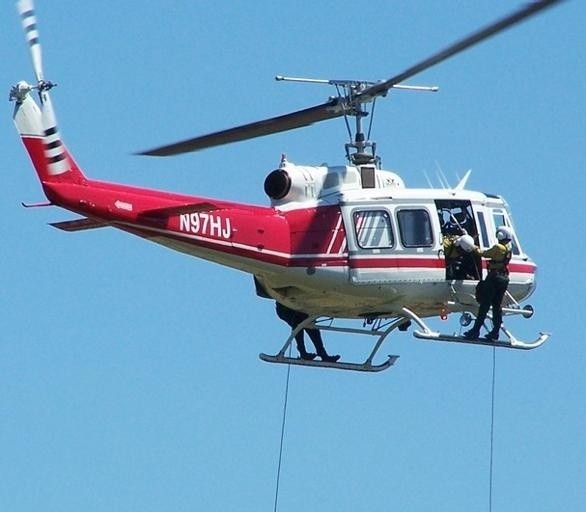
[443,233,481,263]
[275,301,340,362]
[464,226,513,340]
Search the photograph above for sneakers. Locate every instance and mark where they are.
[485,332,499,342]
[463,330,480,337]
[298,343,340,363]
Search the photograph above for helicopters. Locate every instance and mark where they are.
[9,2,565,371]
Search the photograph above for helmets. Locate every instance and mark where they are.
[453,235,474,253]
[496,225,512,242]
